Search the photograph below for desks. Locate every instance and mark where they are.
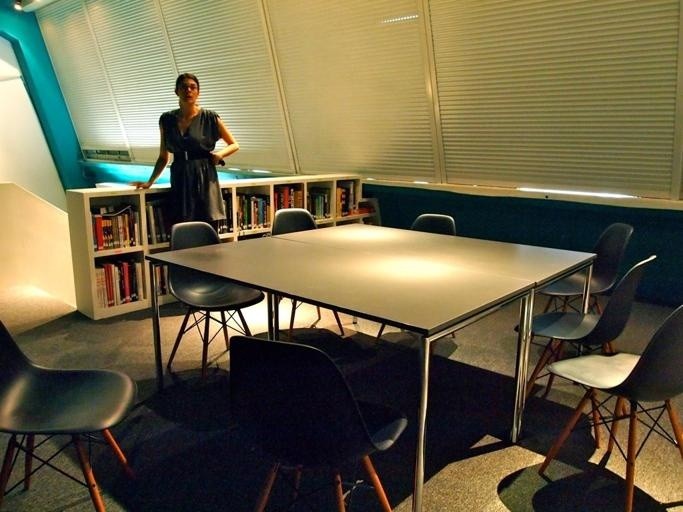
[144,222,598,510]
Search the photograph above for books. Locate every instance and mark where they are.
[92,182,355,308]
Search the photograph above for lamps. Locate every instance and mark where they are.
[14,0,22,11]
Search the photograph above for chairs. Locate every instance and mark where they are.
[514,253,658,419]
[375,211,456,354]
[538,306,683,511]
[228,331,408,511]
[1,319,141,512]
[166,222,266,369]
[535,221,635,351]
[272,210,346,337]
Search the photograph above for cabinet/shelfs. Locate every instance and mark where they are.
[65,173,363,321]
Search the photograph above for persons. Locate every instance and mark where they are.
[130,71,239,233]
